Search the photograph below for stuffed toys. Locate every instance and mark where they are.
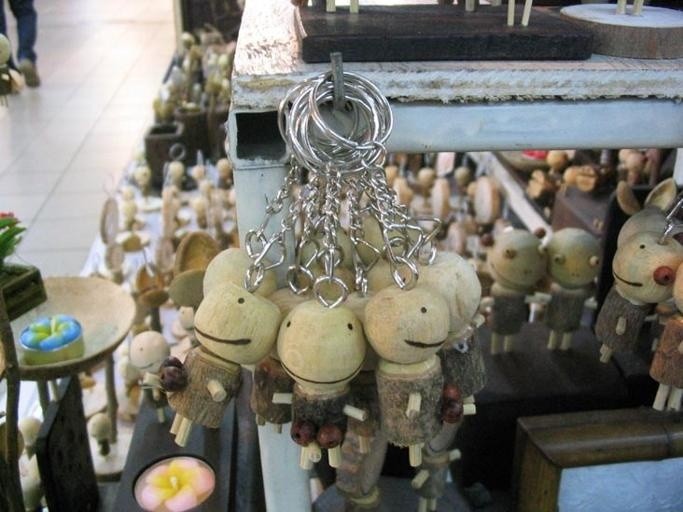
[82,149,682,512]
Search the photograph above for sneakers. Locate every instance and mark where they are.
[19,60,40,88]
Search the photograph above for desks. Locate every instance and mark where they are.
[4,276,138,445]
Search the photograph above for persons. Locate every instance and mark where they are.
[82,149,682,512]
[148,20,233,132]
[0,33,26,109]
[0,0,44,93]
